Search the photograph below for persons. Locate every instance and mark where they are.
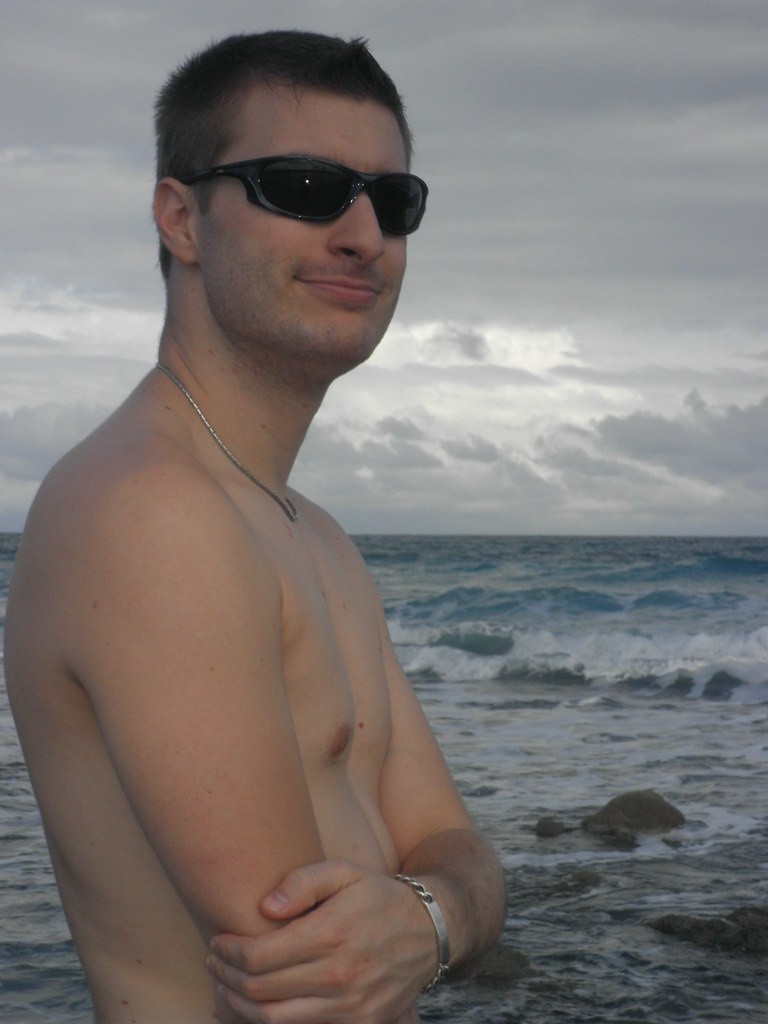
[1,28,510,1024]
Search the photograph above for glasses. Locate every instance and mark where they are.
[175,154,429,236]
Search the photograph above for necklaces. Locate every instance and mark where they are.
[156,362,298,524]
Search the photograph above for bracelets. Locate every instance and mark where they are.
[393,873,450,997]
